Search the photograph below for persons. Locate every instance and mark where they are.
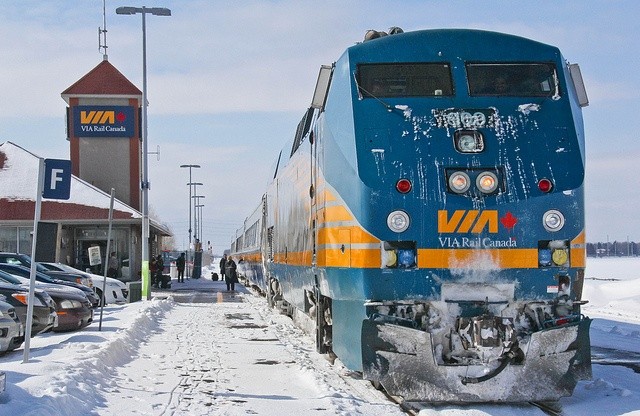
[156,254,165,283]
[151,259,161,270]
[108,252,119,279]
[150,255,162,284]
[223,256,239,292]
[176,252,186,283]
[363,75,450,96]
[492,76,508,94]
[220,254,228,281]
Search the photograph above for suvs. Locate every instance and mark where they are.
[0,276,59,338]
[0,252,93,290]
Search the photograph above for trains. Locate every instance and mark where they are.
[230,27,592,405]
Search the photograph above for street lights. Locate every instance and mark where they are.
[187,183,203,242]
[180,164,200,278]
[116,7,171,301]
[192,196,205,239]
[195,205,204,250]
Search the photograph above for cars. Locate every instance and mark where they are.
[0,301,24,352]
[0,263,100,309]
[16,269,94,332]
[37,262,129,307]
